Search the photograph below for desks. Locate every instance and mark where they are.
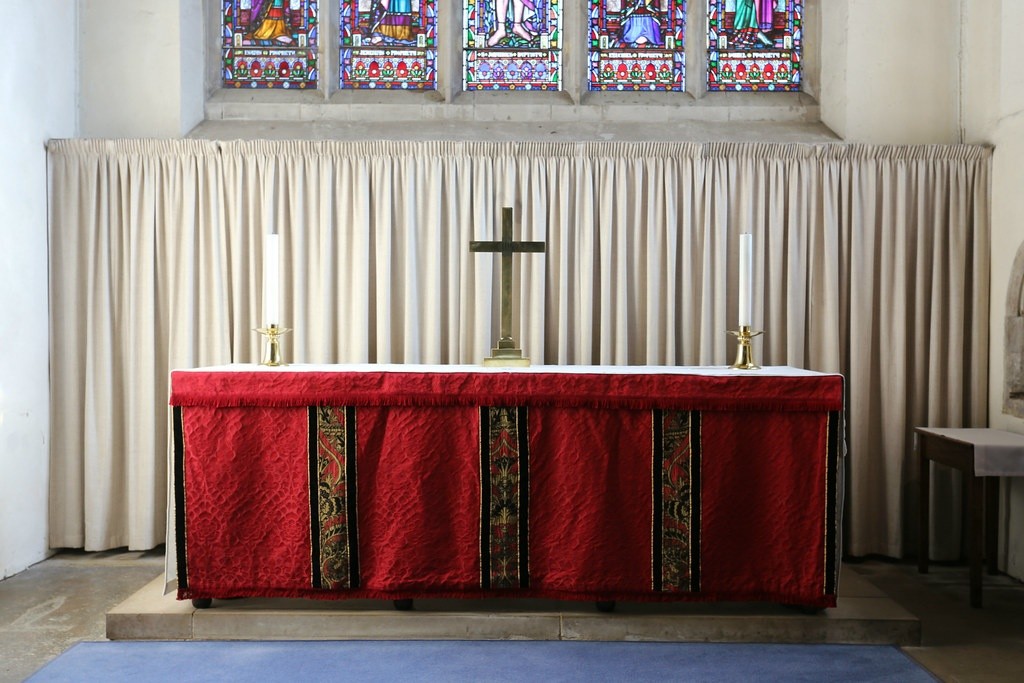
[161,360,845,612]
[917,427,1024,607]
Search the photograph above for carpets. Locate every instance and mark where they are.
[20,643,943,683]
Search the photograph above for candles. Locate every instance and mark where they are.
[737,233,754,329]
[264,232,281,327]
